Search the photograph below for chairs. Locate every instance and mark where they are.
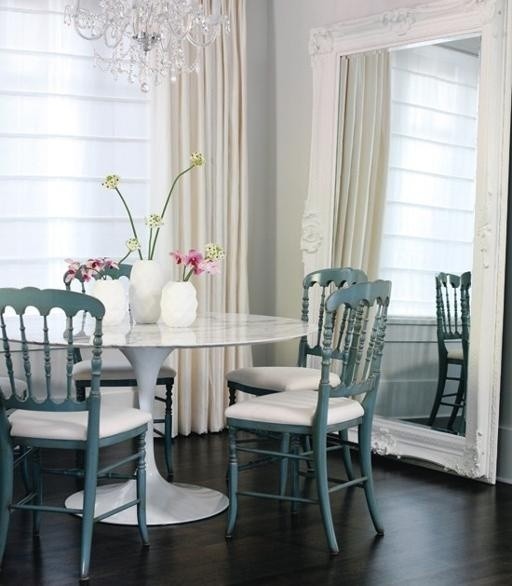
[221,267,369,515]
[224,279,392,557]
[428,269,470,434]
[0,287,151,586]
[73,346,178,479]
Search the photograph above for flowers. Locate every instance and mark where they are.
[62,148,203,285]
[169,243,226,281]
[63,239,141,283]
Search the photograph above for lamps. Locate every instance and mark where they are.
[59,1,231,91]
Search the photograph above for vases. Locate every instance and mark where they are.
[160,280,199,330]
[83,275,132,346]
[129,258,163,325]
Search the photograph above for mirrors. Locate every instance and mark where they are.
[301,0,510,485]
